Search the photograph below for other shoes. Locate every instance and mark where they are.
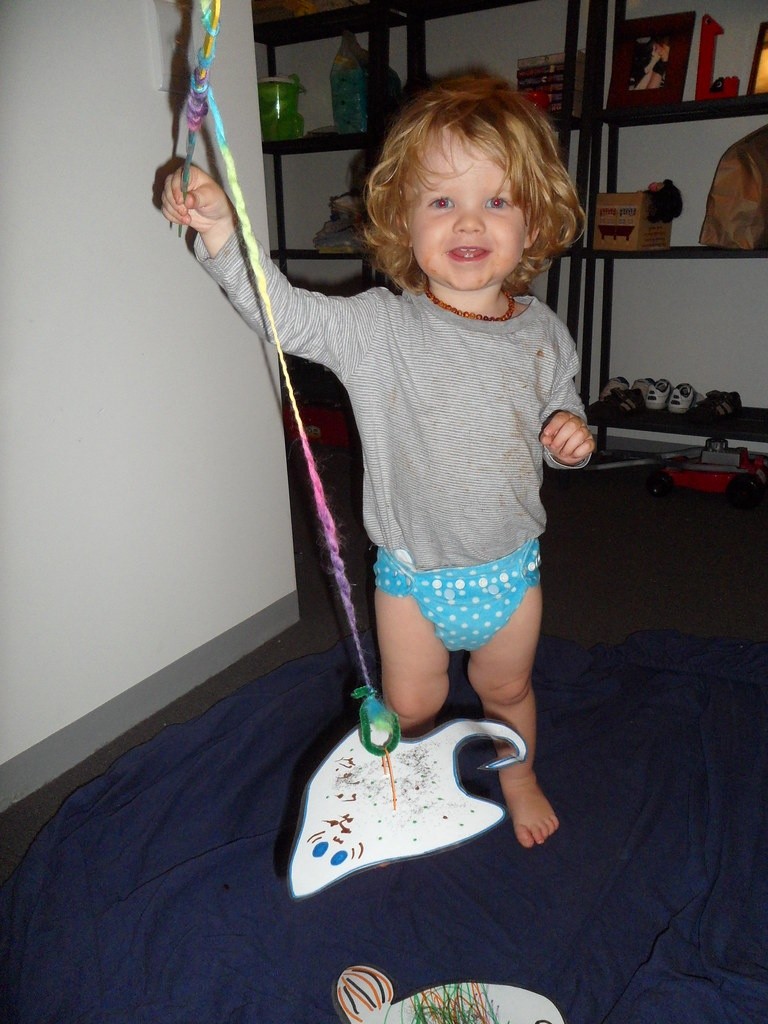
[632,377,654,400]
[601,377,628,401]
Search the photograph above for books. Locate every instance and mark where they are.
[517,50,585,70]
[517,75,585,119]
[516,62,587,77]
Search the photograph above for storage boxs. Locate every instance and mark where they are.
[594,192,673,249]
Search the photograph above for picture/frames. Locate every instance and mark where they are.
[606,10,696,107]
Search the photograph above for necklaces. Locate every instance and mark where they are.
[428,283,516,324]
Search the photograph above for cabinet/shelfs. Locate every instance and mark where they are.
[255,0,768,459]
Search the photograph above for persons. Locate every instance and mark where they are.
[647,33,671,88]
[158,74,596,851]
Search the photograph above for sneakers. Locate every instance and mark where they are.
[688,390,741,422]
[646,378,674,409]
[589,389,644,418]
[668,383,704,414]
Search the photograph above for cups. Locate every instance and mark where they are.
[258,73,307,141]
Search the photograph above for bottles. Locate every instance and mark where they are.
[329,21,369,134]
